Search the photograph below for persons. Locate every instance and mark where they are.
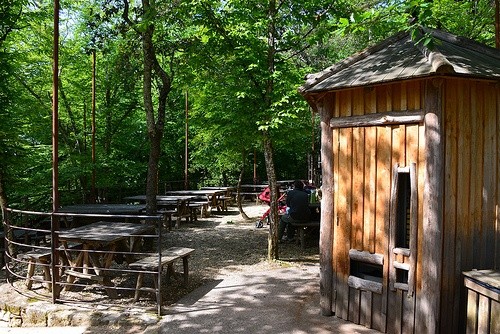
[278,180,316,243]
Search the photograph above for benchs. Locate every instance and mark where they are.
[155,211,178,231]
[186,200,210,218]
[129,246,195,305]
[183,204,202,221]
[25,243,82,292]
[219,197,231,212]
[232,193,261,206]
[291,222,320,250]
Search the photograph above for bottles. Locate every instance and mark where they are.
[311,189,316,203]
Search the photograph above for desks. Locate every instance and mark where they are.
[201,187,234,206]
[240,185,269,191]
[307,202,320,208]
[58,221,155,299]
[166,190,225,213]
[276,180,295,185]
[124,195,196,220]
[56,204,148,213]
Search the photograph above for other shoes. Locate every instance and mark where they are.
[288,238,295,241]
[278,240,281,243]
